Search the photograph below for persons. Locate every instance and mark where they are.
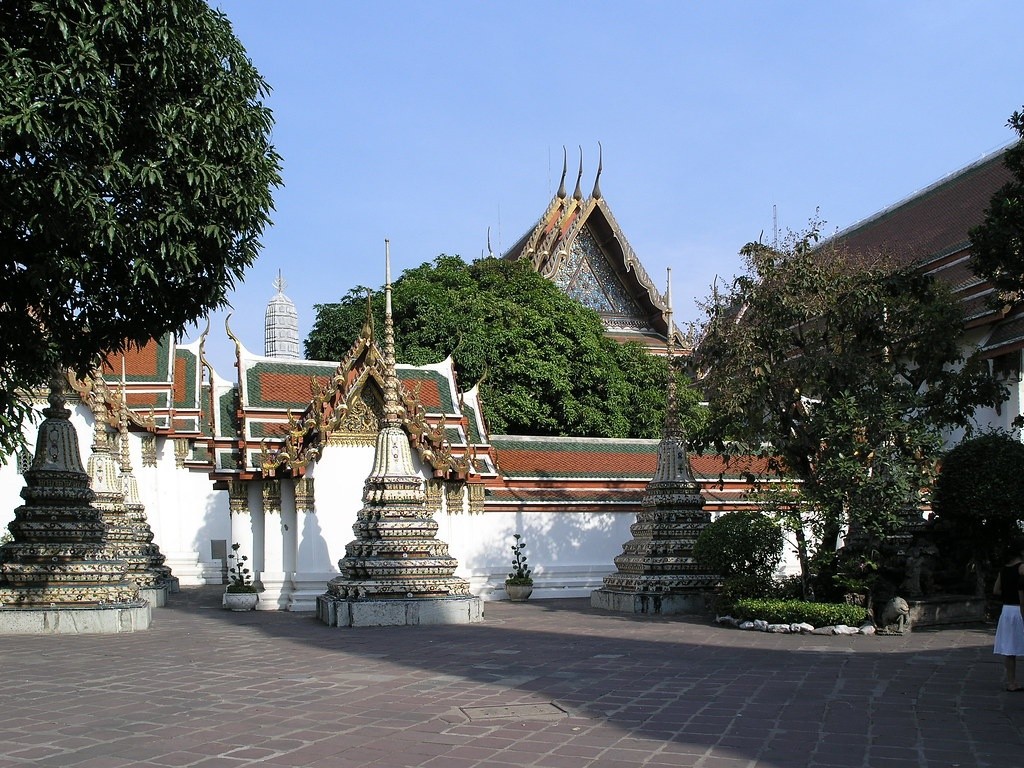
[992,540,1024,691]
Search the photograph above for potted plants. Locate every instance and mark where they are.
[225,544,257,611]
[505,533,534,601]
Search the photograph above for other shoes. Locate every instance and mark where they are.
[1007,685,1024,692]
